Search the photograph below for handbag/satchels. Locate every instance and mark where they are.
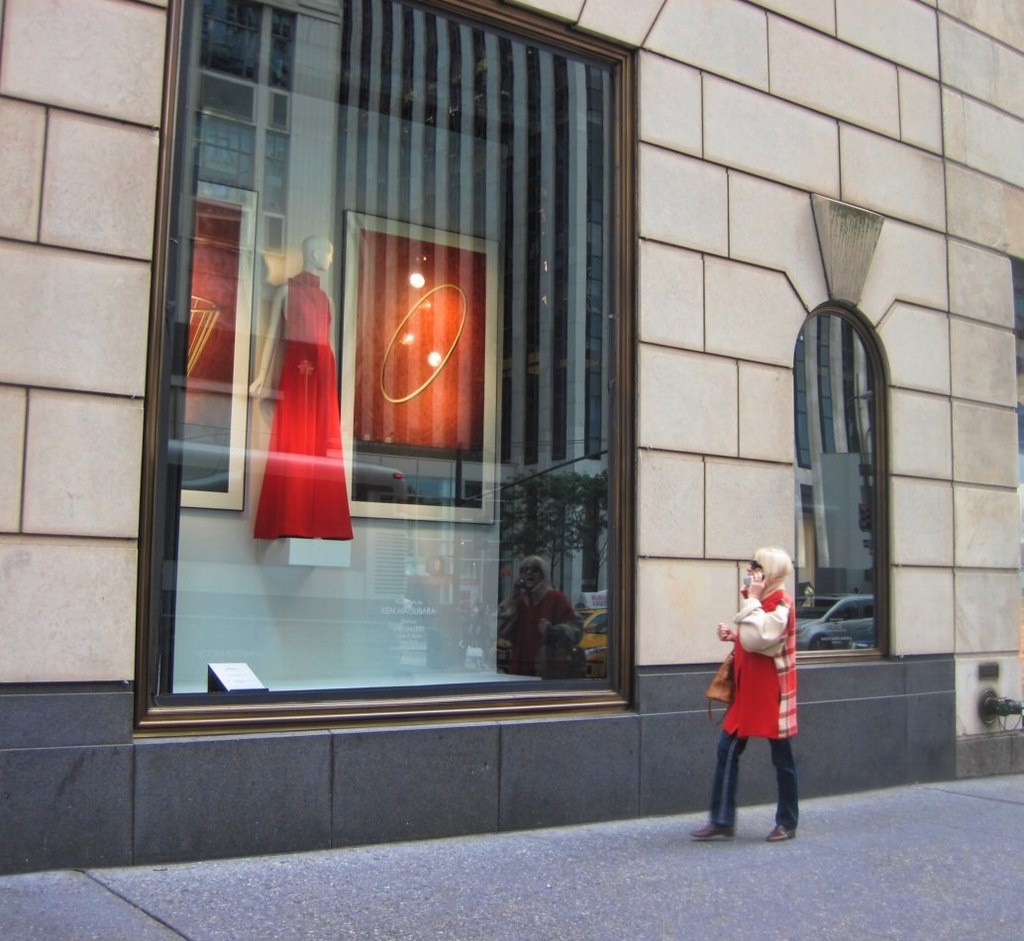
[705,648,735,726]
[534,642,586,679]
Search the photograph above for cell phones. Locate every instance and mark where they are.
[516,576,524,587]
[743,574,765,587]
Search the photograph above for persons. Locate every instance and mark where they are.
[802,582,816,608]
[496,555,583,679]
[691,548,799,841]
[248,236,354,538]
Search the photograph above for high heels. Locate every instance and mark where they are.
[690,822,734,837]
[767,824,796,841]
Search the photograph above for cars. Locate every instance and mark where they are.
[489,608,608,679]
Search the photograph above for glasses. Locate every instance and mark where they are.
[748,559,763,572]
[520,565,541,575]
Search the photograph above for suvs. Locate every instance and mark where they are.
[795,594,876,650]
